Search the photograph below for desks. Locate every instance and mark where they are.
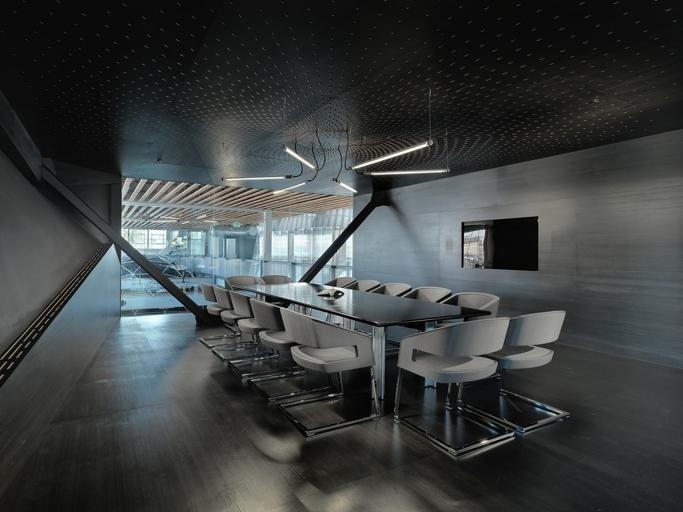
[234,281,495,404]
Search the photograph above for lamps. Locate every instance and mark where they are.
[221,88,452,198]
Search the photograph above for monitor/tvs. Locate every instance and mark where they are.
[460,216,539,272]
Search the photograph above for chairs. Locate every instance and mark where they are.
[210,285,253,362]
[399,284,453,304]
[448,308,570,433]
[218,290,258,363]
[393,315,518,454]
[367,282,413,298]
[341,278,382,293]
[276,308,382,437]
[240,297,328,401]
[225,274,263,292]
[438,290,501,316]
[257,273,295,284]
[199,280,245,350]
[322,276,356,288]
